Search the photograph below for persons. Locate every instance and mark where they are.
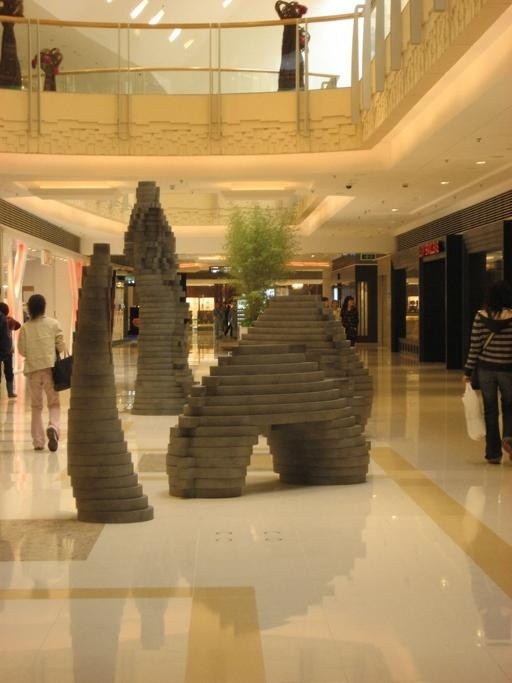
[18,294,65,452]
[222,302,234,338]
[331,298,342,322]
[464,277,511,465]
[340,294,359,346]
[211,300,226,340]
[0,302,22,398]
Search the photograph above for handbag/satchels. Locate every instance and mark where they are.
[52,340,75,392]
[469,365,481,391]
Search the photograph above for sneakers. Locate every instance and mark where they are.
[33,425,59,452]
[8,392,17,397]
[485,436,512,464]
